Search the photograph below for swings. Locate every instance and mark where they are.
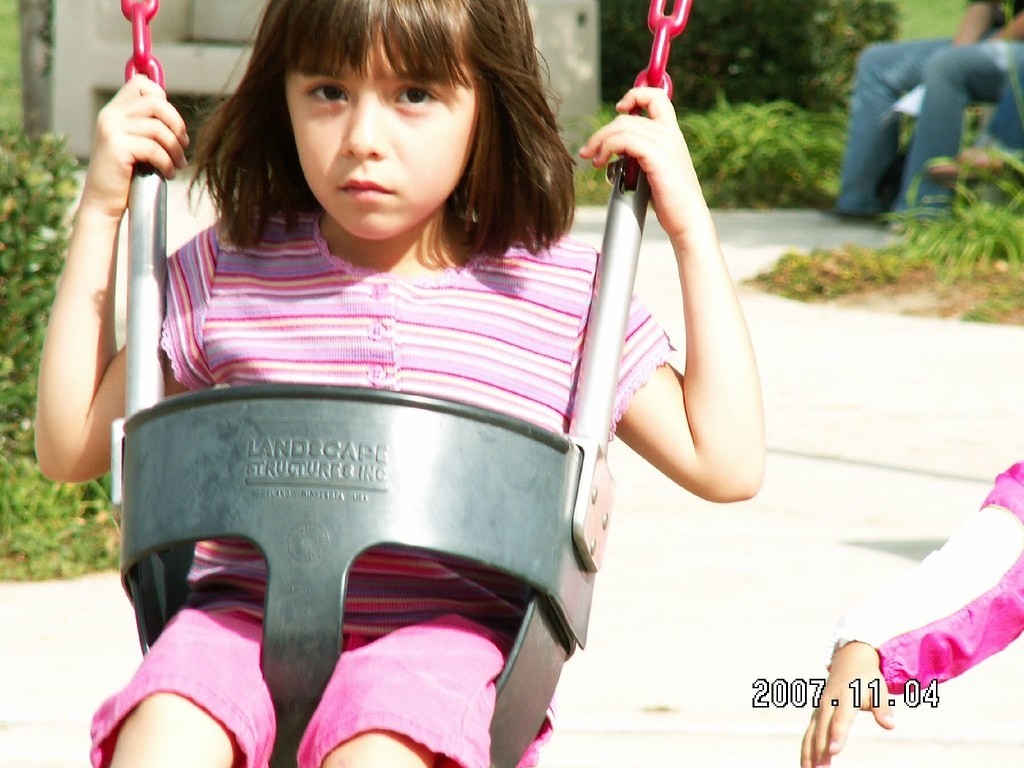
[122,0,690,768]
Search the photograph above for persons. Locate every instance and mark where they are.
[801,461,1024,767]
[34,0,769,767]
[831,0,1024,235]
